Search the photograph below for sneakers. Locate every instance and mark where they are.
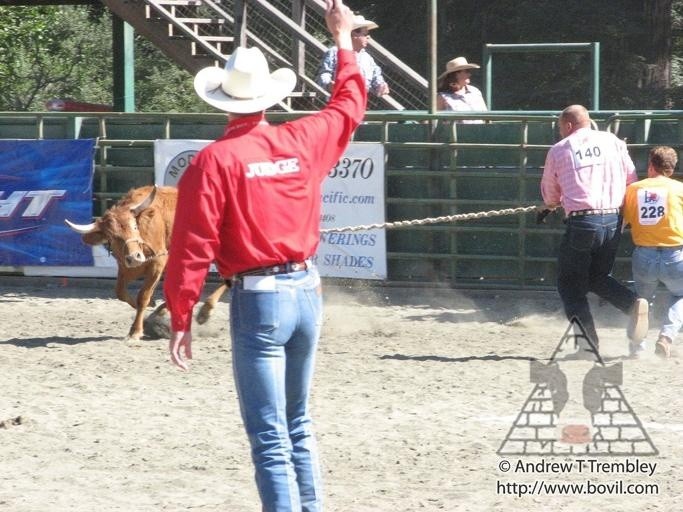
[566,347,599,361]
[630,298,670,359]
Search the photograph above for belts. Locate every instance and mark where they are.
[567,208,618,216]
[239,259,306,275]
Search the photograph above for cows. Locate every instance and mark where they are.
[64,184,229,349]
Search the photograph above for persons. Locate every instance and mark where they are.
[436,55,488,124]
[624,145,683,358]
[164,2,367,512]
[540,104,650,361]
[314,15,389,106]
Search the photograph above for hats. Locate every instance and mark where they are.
[193,46,298,114]
[438,56,480,81]
[350,15,378,31]
